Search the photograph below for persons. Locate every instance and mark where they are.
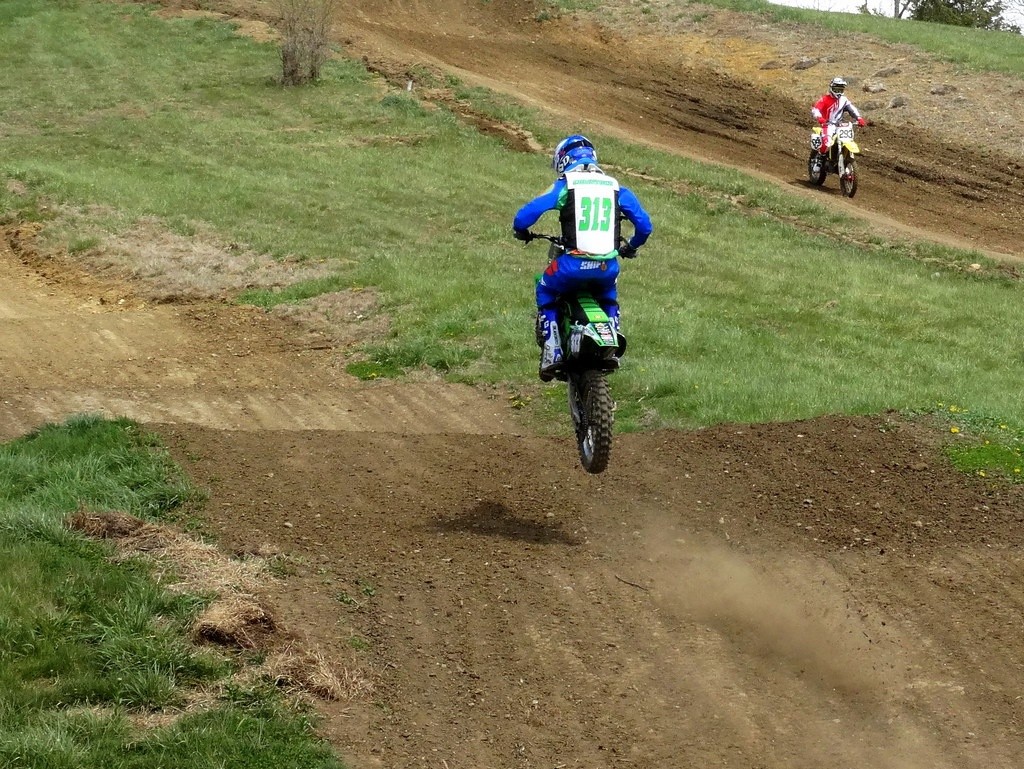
[811,78,865,172]
[512,135,653,382]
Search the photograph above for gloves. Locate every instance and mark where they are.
[513,226,533,245]
[856,116,866,127]
[619,241,637,259]
[817,117,827,125]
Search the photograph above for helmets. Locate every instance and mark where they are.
[830,78,847,99]
[553,135,597,176]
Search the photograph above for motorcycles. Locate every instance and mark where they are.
[513,229,637,474]
[807,121,862,198]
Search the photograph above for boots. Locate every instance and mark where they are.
[603,356,621,373]
[538,321,563,382]
[813,150,826,172]
